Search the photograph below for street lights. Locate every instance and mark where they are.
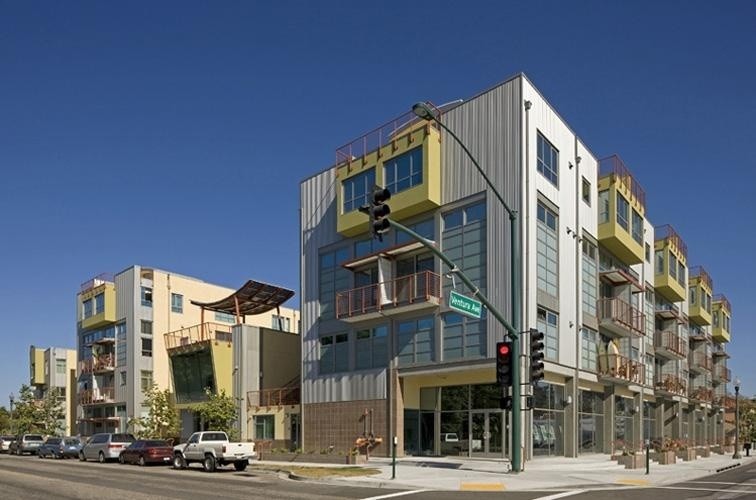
[411,100,526,476]
[8,391,15,433]
[732,373,742,460]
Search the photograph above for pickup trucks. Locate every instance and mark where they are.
[171,429,257,473]
[439,432,482,452]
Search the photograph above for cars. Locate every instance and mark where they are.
[0,432,174,467]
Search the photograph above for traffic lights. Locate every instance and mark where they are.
[529,398,536,408]
[495,341,512,387]
[369,184,391,242]
[500,398,509,408]
[528,327,545,383]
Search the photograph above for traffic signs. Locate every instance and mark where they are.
[447,290,482,320]
